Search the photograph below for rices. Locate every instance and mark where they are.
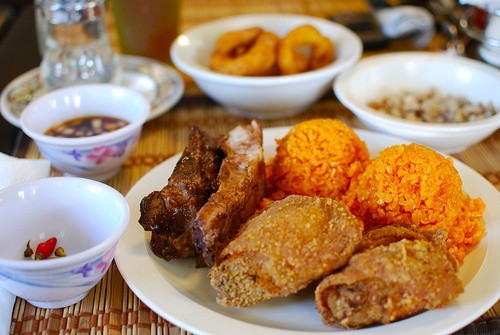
[266,116,363,212]
[357,136,484,260]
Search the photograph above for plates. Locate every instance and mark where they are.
[0,53,184,127]
[113,126,500,334]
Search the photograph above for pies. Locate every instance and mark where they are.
[275,22,340,75]
[209,26,275,79]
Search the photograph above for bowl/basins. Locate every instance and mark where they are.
[0,177,130,308]
[21,85,151,182]
[170,13,363,120]
[333,51,500,155]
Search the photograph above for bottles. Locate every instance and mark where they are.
[35,0,114,89]
